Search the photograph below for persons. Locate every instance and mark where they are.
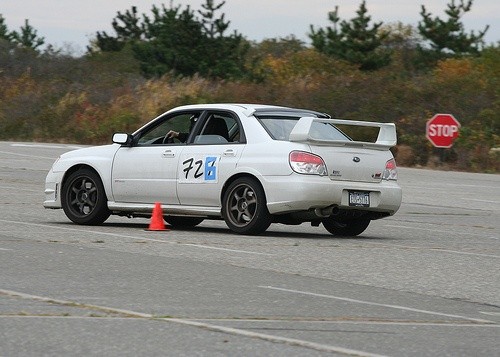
[165,113,201,143]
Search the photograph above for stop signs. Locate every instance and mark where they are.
[426,114,461,148]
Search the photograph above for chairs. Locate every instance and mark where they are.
[189,117,231,142]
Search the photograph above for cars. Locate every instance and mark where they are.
[43,103,402,237]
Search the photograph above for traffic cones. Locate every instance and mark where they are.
[145,202,171,231]
